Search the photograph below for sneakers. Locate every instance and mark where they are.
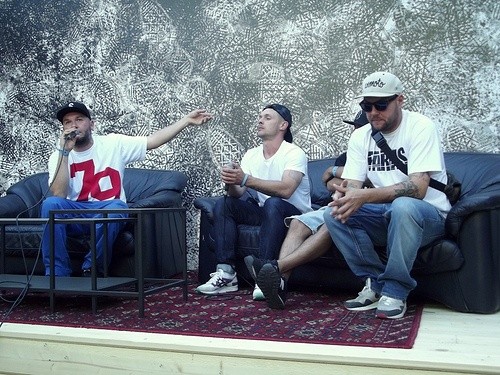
[256,263,287,309]
[252,281,267,301]
[194,269,239,295]
[375,296,408,319]
[343,277,382,311]
[244,255,280,283]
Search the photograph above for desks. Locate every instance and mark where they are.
[0,207,189,317]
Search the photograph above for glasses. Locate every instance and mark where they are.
[359,94,398,112]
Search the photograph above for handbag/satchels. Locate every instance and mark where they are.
[443,172,462,206]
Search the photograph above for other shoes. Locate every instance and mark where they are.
[82,269,92,278]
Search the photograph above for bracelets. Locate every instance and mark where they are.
[333,166,339,177]
[58,148,69,156]
[240,174,249,188]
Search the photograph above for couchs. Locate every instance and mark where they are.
[0,168,190,279]
[194,153,500,314]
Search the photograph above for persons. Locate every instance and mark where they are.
[324,70,453,319]
[245,109,370,308]
[42,101,213,309]
[197,103,313,298]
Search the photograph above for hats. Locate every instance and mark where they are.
[56,102,91,123]
[356,71,403,98]
[263,104,293,143]
[343,110,369,127]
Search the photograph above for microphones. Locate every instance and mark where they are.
[66,130,79,139]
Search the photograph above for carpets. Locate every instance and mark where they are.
[0,274,424,349]
